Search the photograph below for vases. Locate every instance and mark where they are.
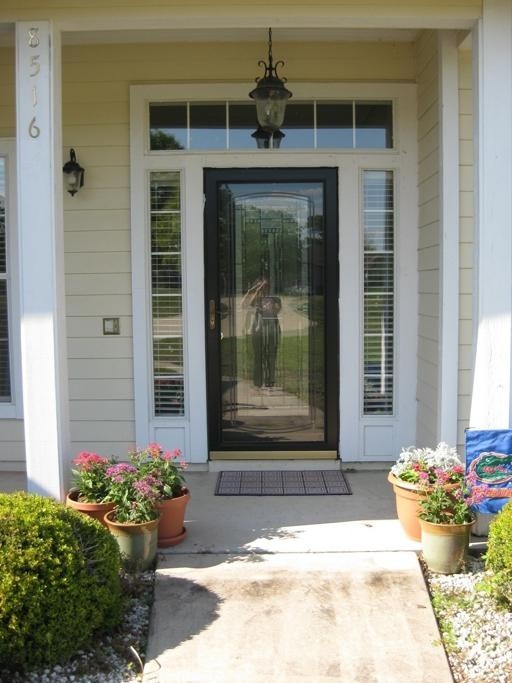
[387,471,478,574]
[66,486,190,572]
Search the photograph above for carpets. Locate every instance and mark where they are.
[214,471,354,496]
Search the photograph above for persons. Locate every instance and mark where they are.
[242,277,280,388]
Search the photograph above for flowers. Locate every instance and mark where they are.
[391,442,489,526]
[70,442,188,523]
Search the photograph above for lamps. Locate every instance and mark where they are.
[248,26,292,134]
[252,125,285,149]
[62,148,84,198]
